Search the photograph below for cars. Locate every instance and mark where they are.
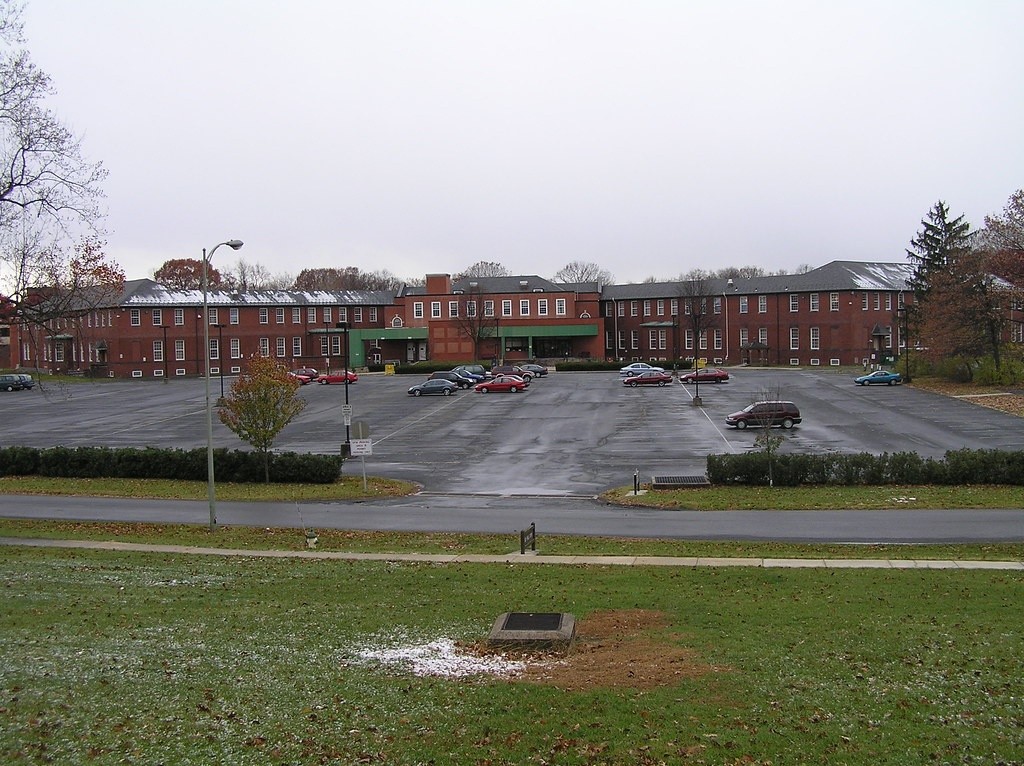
[502,375,530,387]
[428,371,474,390]
[475,377,526,394]
[854,371,901,386]
[680,369,729,385]
[0,374,35,392]
[619,362,673,387]
[287,368,358,385]
[725,401,802,429]
[520,364,549,378]
[408,379,459,397]
[451,365,486,384]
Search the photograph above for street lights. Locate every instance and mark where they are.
[202,240,245,530]
[335,322,349,443]
[323,322,332,376]
[160,324,227,398]
[671,315,702,398]
[494,318,500,365]
[897,309,909,379]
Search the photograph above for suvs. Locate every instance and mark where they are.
[491,365,536,383]
